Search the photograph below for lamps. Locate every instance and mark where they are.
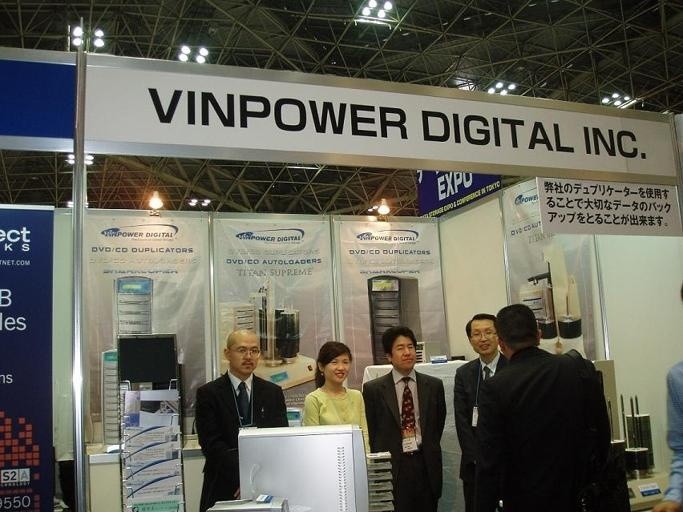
[378,198,391,216]
[149,190,163,210]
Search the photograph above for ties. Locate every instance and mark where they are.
[483,365,492,381]
[238,381,252,427]
[402,376,416,430]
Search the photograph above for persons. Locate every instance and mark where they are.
[468,304,632,511]
[452,311,513,511]
[194,327,290,510]
[299,342,369,464]
[644,280,682,512]
[360,326,447,511]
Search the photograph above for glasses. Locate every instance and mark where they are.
[230,347,261,358]
[469,330,498,338]
